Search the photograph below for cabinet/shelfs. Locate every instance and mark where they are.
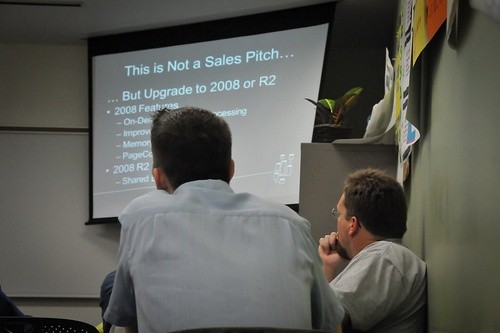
[300,142,399,235]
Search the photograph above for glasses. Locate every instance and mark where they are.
[331,207,363,230]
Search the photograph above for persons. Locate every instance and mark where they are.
[317,167,429,333]
[103,106,345,333]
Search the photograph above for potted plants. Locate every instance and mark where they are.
[306,87,363,143]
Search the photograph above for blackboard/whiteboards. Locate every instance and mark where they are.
[0,127,122,302]
